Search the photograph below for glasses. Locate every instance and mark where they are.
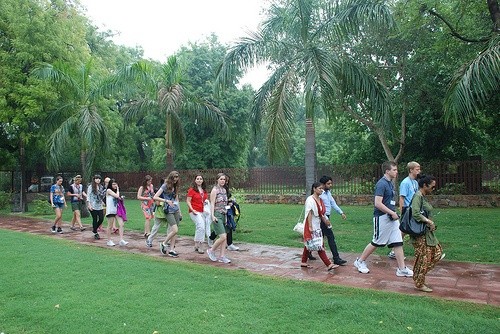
[427,184,436,191]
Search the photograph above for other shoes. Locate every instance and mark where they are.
[194,243,205,254]
[415,285,432,291]
[334,259,347,265]
[308,254,316,260]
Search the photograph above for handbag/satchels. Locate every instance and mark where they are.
[293,223,304,235]
[156,202,167,219]
[399,194,428,237]
[164,209,169,214]
[304,229,325,252]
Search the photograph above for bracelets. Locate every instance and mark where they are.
[192,209,194,212]
[165,199,167,202]
[429,221,434,225]
[426,218,429,223]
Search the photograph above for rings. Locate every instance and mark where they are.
[433,228,434,230]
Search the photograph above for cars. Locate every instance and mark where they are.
[28,176,55,193]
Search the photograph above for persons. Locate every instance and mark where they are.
[301,183,339,271]
[308,176,347,265]
[146,177,176,248]
[206,173,239,263]
[87,175,119,239]
[153,171,182,257]
[105,179,129,246]
[50,176,67,233]
[137,175,155,238]
[187,174,208,254]
[409,171,443,292]
[389,161,446,262]
[68,175,88,231]
[352,161,414,277]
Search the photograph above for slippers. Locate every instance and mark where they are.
[301,263,313,268]
[327,264,339,271]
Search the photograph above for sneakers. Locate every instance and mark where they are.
[227,244,239,251]
[388,251,406,260]
[353,257,370,274]
[396,266,414,277]
[208,237,214,248]
[160,242,167,255]
[207,249,217,262]
[167,243,175,248]
[218,256,231,264]
[168,251,179,258]
[49,225,153,248]
